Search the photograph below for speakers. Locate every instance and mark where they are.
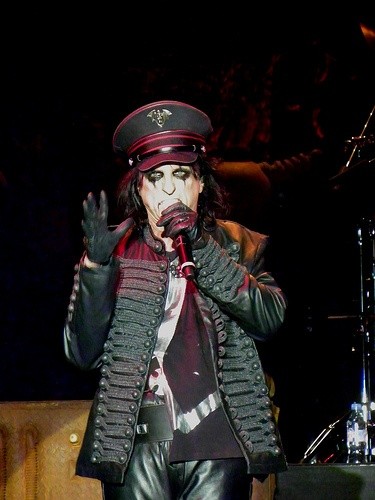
[278,463,375,500]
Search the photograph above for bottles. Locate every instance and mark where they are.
[347,403,367,463]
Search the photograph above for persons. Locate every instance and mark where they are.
[64,100,287,500]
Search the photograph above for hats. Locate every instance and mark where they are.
[112,100,214,171]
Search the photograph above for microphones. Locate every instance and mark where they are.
[166,208,195,279]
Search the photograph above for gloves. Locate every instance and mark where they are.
[157,202,211,250]
[80,190,134,265]
